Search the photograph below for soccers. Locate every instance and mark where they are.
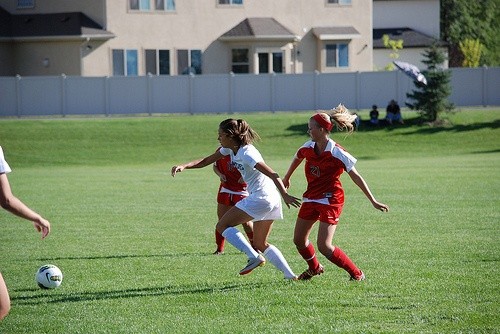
[34,264,63,289]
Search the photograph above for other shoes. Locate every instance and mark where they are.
[253,246,261,254]
[213,251,224,255]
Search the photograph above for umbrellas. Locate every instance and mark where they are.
[392,60,427,86]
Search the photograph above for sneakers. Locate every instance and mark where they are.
[349,270,366,281]
[284,275,298,280]
[298,264,325,280]
[239,254,265,275]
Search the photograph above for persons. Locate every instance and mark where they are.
[370,105,379,124]
[172,118,302,280]
[0,146,50,319]
[386,100,404,125]
[282,104,389,281]
[349,114,358,131]
[212,147,261,255]
